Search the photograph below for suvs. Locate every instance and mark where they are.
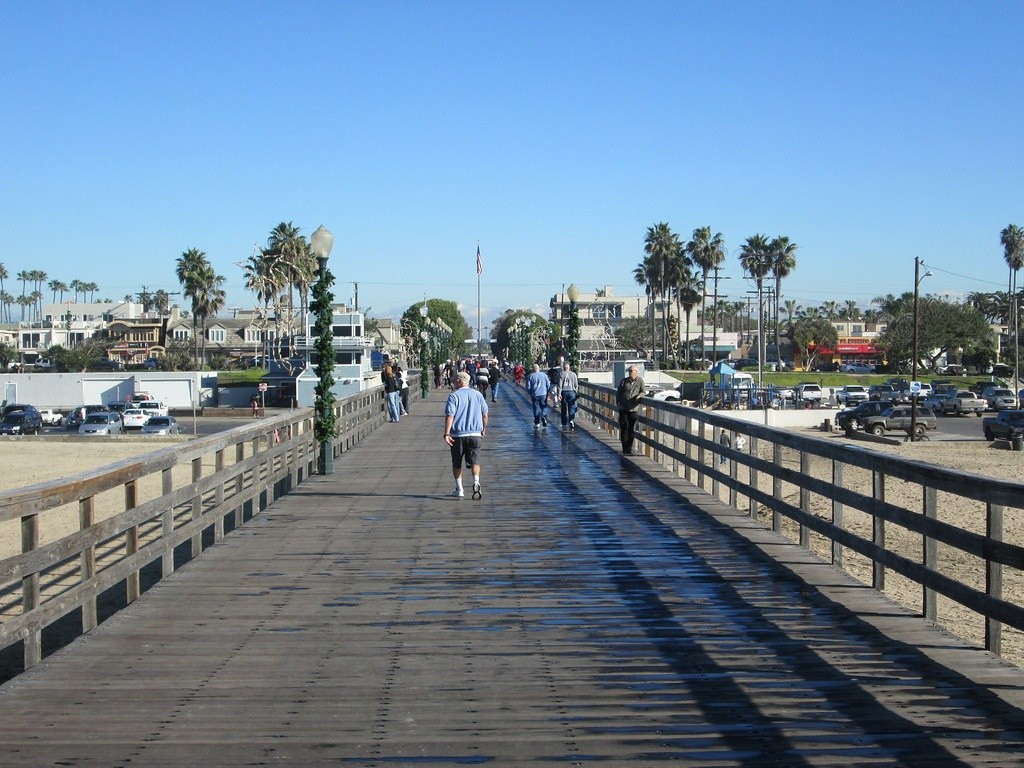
[130,393,154,409]
[862,406,937,437]
[835,401,895,430]
[144,358,162,369]
[643,386,681,404]
[34,357,56,368]
[93,358,123,371]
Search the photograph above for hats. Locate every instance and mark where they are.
[451,372,470,383]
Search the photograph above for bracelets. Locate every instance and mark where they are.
[444,434,451,438]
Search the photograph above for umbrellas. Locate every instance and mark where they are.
[708,363,736,375]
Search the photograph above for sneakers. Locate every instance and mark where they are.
[472,483,482,500]
[452,487,465,497]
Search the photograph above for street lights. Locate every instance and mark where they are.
[311,224,335,475]
[508,314,536,387]
[911,272,935,440]
[420,305,430,398]
[425,316,452,388]
[567,283,580,370]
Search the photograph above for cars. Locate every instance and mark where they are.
[141,415,180,435]
[728,373,1024,417]
[811,362,1013,378]
[65,401,125,430]
[691,358,797,371]
[123,409,151,428]
[226,355,268,366]
[39,410,63,425]
[139,400,168,416]
[78,411,123,436]
[0,402,42,436]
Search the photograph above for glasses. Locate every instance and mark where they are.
[629,370,637,372]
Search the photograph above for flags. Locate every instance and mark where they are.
[477,246,482,274]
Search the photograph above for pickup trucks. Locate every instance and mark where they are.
[982,410,1024,441]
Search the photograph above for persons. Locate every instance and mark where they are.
[870,393,898,405]
[382,357,523,421]
[526,364,550,428]
[615,366,645,453]
[548,361,564,407]
[443,371,488,499]
[558,362,578,428]
[252,400,258,419]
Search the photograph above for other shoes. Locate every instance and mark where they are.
[390,418,399,423]
[562,424,568,428]
[401,412,408,417]
[541,417,547,427]
[569,421,574,428]
[534,423,540,428]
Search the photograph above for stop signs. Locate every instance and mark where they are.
[259,383,268,391]
